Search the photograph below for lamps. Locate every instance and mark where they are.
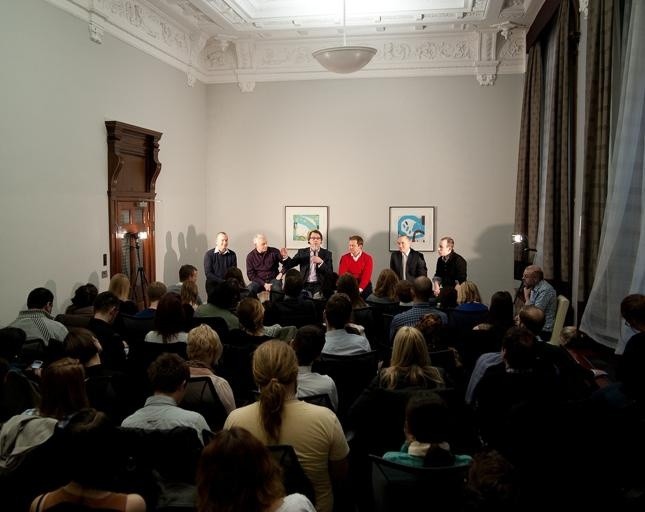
[311,0,378,73]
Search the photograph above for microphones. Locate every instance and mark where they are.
[313,246,317,255]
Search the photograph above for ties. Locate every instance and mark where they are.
[311,248,317,256]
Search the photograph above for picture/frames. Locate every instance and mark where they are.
[285,206,328,250]
[389,206,435,252]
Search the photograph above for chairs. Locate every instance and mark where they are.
[0,273,645,512]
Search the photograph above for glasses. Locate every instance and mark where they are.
[310,237,321,240]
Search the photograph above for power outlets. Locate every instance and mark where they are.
[102,271,107,277]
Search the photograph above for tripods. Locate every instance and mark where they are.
[133,269,150,308]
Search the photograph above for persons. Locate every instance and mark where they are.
[0,263,644,510]
[244,234,287,305]
[390,235,427,281]
[337,236,372,301]
[428,236,467,305]
[204,231,238,307]
[280,231,333,299]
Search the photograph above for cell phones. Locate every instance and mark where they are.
[32,360,43,370]
